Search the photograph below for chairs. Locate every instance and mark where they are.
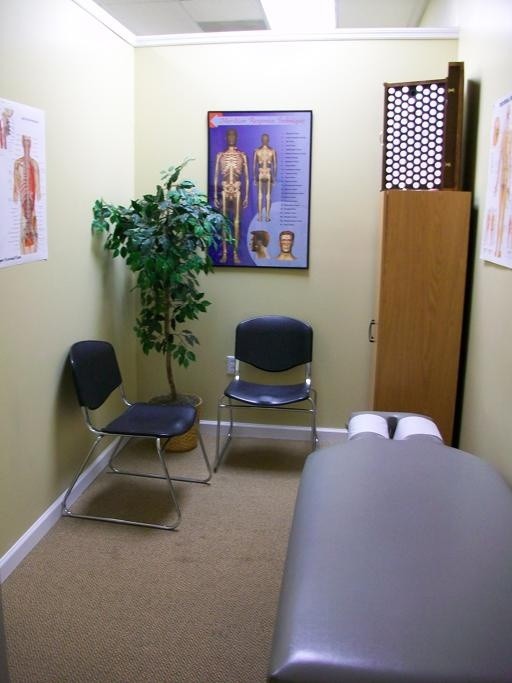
[61,341,212,532]
[213,315,316,472]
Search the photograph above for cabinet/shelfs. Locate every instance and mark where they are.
[367,190,472,446]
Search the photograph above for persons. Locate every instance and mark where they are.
[249,229,271,259]
[273,230,299,262]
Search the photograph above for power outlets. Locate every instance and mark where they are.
[226,355,236,375]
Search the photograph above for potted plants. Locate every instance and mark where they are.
[91,156,239,454]
